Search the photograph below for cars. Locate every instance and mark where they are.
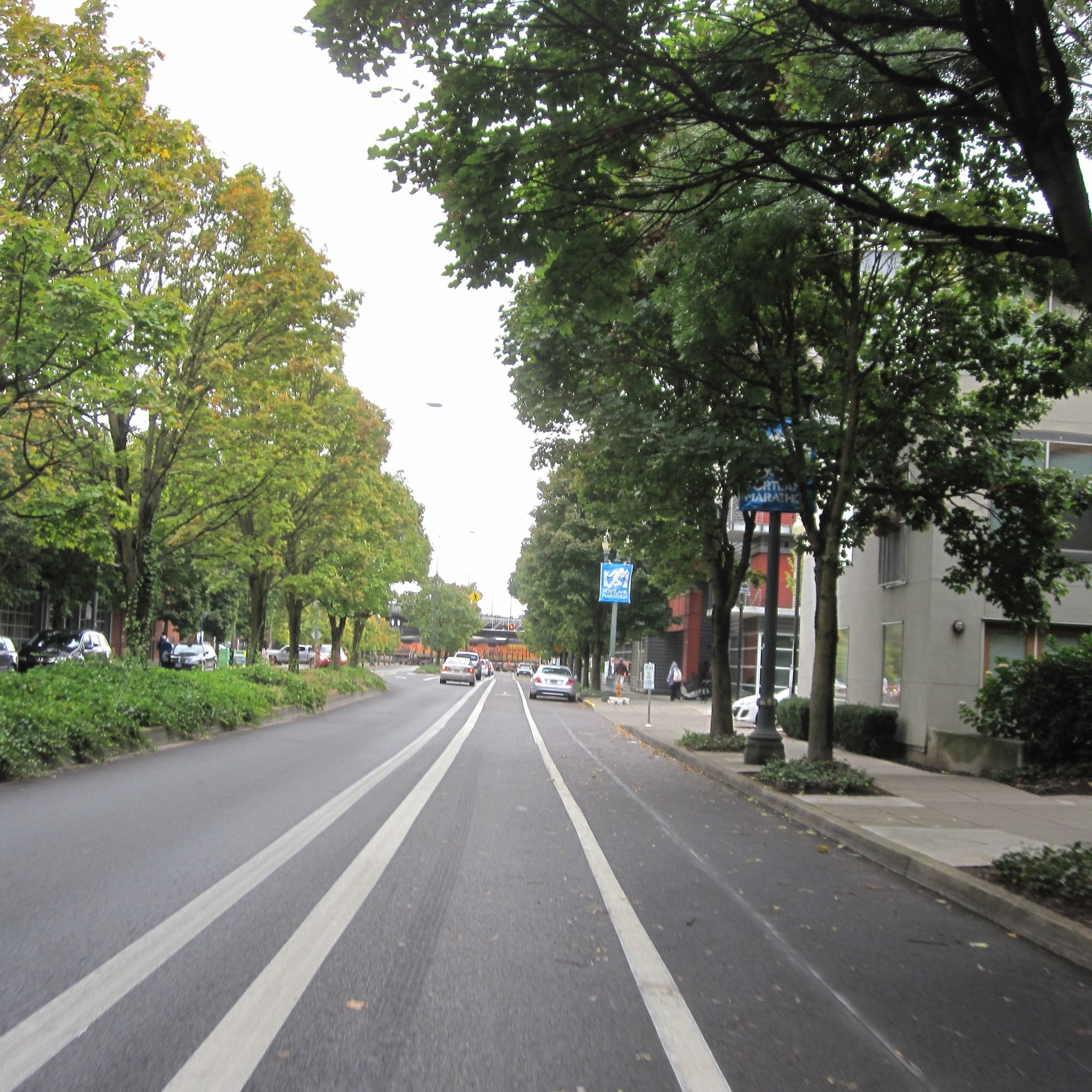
[165,643,348,671]
[441,651,577,701]
[730,681,847,729]
[0,629,112,675]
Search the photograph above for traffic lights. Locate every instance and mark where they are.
[507,617,515,631]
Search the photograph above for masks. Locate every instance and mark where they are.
[162,635,166,639]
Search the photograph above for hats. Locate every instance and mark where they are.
[619,658,623,661]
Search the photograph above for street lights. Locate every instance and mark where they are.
[601,529,633,703]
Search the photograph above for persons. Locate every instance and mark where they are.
[157,635,172,666]
[613,658,629,691]
[666,661,683,702]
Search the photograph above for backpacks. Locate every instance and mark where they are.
[673,667,682,681]
